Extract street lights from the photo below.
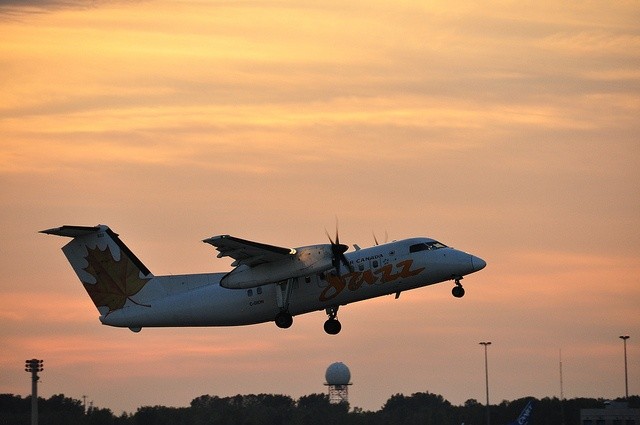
[618,334,631,400]
[478,340,495,425]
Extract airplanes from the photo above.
[37,221,488,337]
